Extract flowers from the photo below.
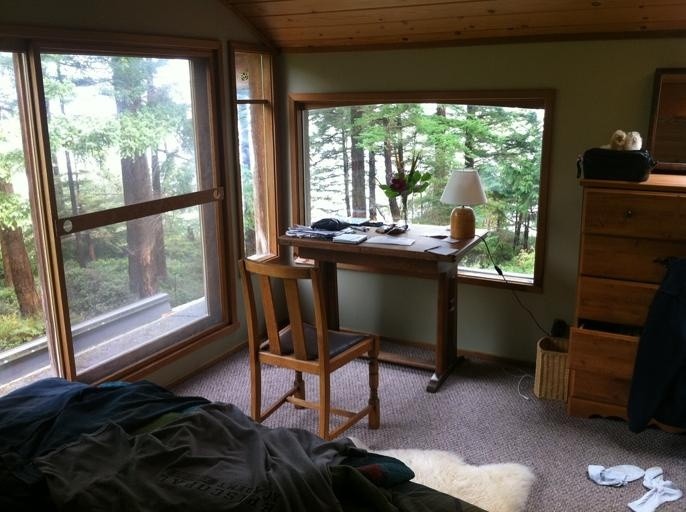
[378,167,432,227]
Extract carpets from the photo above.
[346,437,536,512]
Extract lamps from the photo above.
[439,169,487,241]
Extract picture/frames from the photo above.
[646,65,684,175]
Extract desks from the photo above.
[272,219,488,391]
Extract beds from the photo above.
[0,376,485,510]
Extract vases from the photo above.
[399,200,411,231]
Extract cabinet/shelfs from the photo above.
[563,171,685,435]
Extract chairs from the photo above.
[235,256,380,442]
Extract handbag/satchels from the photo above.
[311,218,349,231]
[577,147,656,182]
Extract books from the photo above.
[333,233,367,245]
[412,238,459,257]
